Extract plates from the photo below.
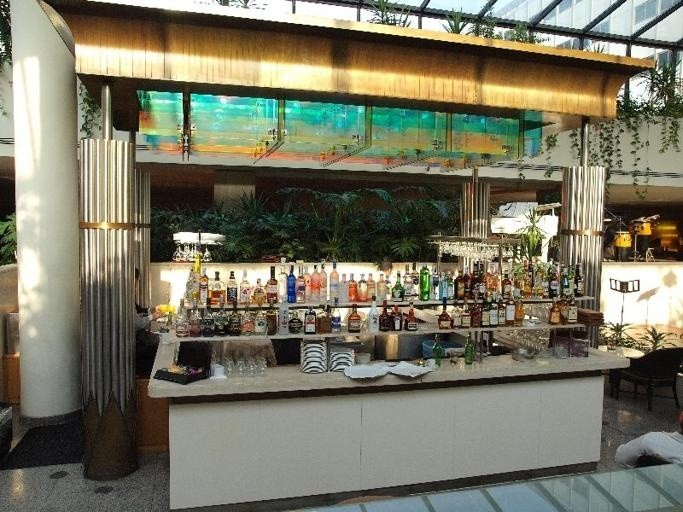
[344,361,432,379]
[300,341,356,374]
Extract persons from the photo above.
[614,413,683,469]
[135,266,164,378]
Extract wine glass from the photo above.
[438,242,513,257]
[172,241,213,262]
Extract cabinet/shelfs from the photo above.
[149,297,630,401]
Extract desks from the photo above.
[597,346,645,398]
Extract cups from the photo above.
[229,355,267,379]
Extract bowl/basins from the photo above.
[356,352,370,363]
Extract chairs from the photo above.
[617,347,683,411]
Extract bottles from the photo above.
[432,333,475,367]
[176,258,583,338]
